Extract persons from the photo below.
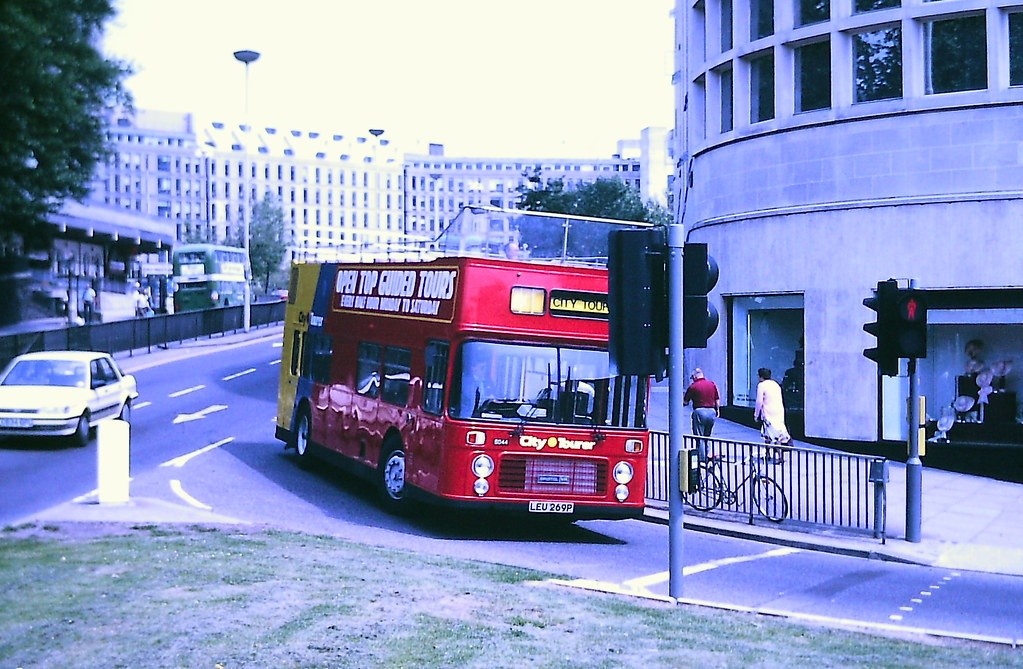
[460,357,499,417]
[81,284,96,326]
[682,368,720,465]
[503,244,521,261]
[754,367,793,464]
[137,286,152,318]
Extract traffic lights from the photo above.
[606,230,665,378]
[684,242,720,347]
[863,278,926,378]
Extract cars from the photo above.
[0,351,138,447]
[276,290,288,301]
[23,271,173,319]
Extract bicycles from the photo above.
[681,451,788,523]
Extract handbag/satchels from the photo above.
[774,436,794,452]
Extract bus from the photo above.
[275,257,650,525]
[174,244,252,307]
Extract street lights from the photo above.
[369,128,384,258]
[233,50,261,333]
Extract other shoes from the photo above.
[698,460,706,463]
[762,456,770,460]
[777,458,784,463]
